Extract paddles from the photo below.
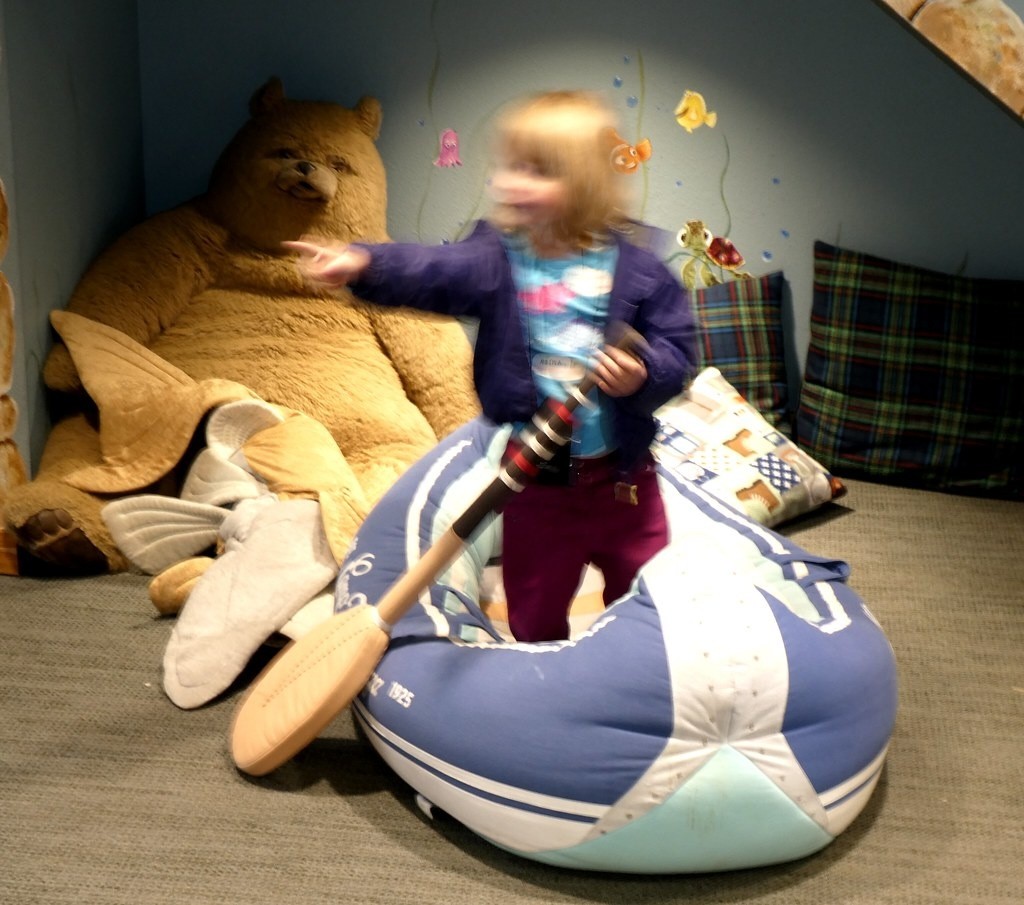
[227,329,655,778]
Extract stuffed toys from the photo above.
[9,81,486,710]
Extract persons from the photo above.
[280,91,700,644]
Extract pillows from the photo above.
[650,363,846,531]
[794,240,1024,502]
[676,272,792,444]
